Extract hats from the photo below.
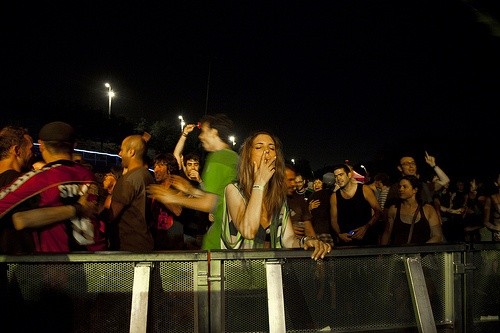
[323,172,337,185]
[37,121,76,144]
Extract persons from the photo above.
[0,112,241,333]
[219,133,331,333]
[284,151,500,333]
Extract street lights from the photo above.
[361,165,368,182]
[105,82,119,144]
[178,115,186,133]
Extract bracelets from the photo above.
[432,165,437,168]
[303,235,316,240]
[252,185,264,191]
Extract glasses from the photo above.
[401,160,416,166]
